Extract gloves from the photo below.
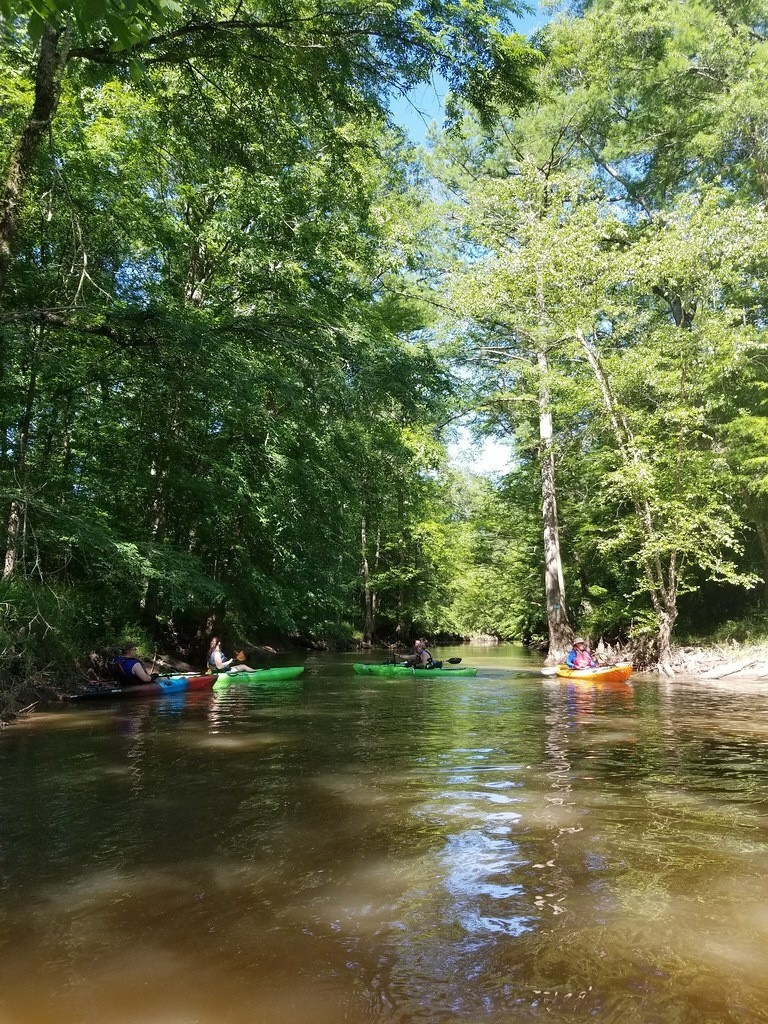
[149,673,159,682]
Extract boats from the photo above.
[159,665,307,683]
[352,662,479,678]
[68,674,220,702]
[556,661,636,682]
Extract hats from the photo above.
[573,638,585,644]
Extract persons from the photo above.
[207,636,256,673]
[113,642,159,685]
[565,637,600,670]
[393,638,432,669]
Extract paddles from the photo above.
[158,672,204,678]
[233,650,248,662]
[538,661,634,676]
[435,657,463,664]
